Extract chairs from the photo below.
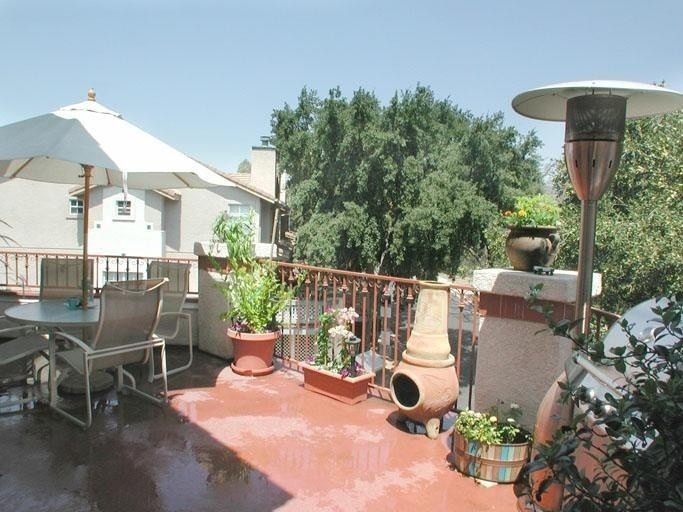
[0,259,195,429]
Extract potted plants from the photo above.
[452,395,535,485]
[212,206,312,379]
[298,307,377,407]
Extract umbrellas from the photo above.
[0,87,240,342]
